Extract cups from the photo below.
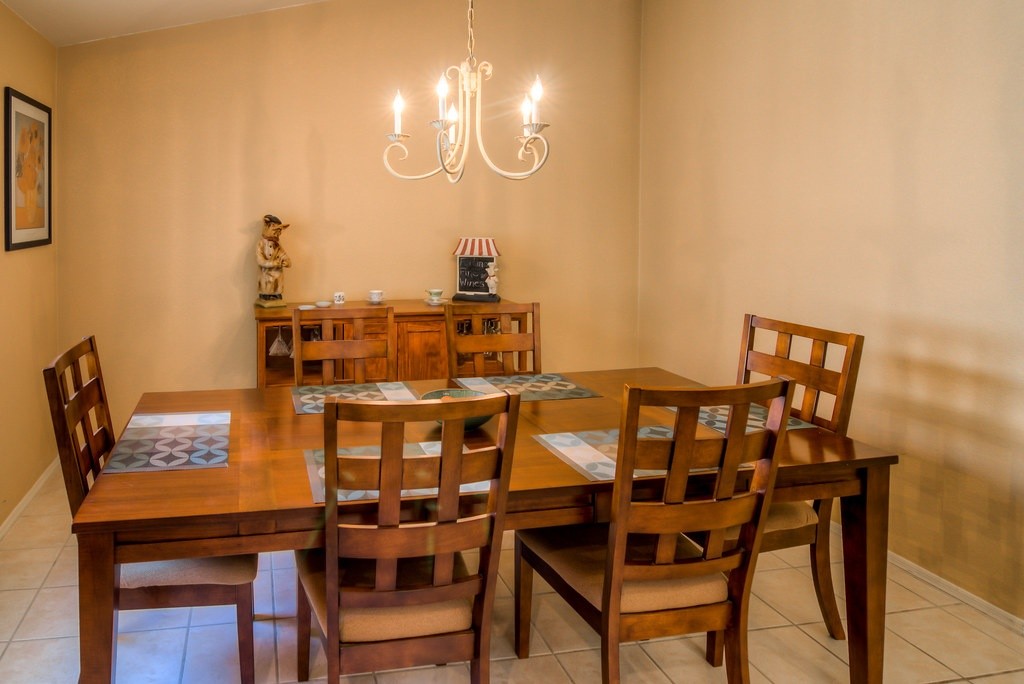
[429,289,443,302]
[370,290,383,300]
[334,291,345,303]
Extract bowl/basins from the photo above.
[420,389,495,429]
[316,301,332,307]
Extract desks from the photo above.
[72,362,899,683]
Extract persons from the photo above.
[256,215,291,301]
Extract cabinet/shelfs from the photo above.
[255,295,528,388]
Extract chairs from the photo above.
[291,306,396,386]
[706,312,864,669]
[515,373,796,682]
[43,333,257,683]
[295,386,521,682]
[444,303,542,377]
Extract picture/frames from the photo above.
[3,85,52,252]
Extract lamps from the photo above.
[384,0,558,182]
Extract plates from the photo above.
[425,299,448,306]
[369,300,383,304]
